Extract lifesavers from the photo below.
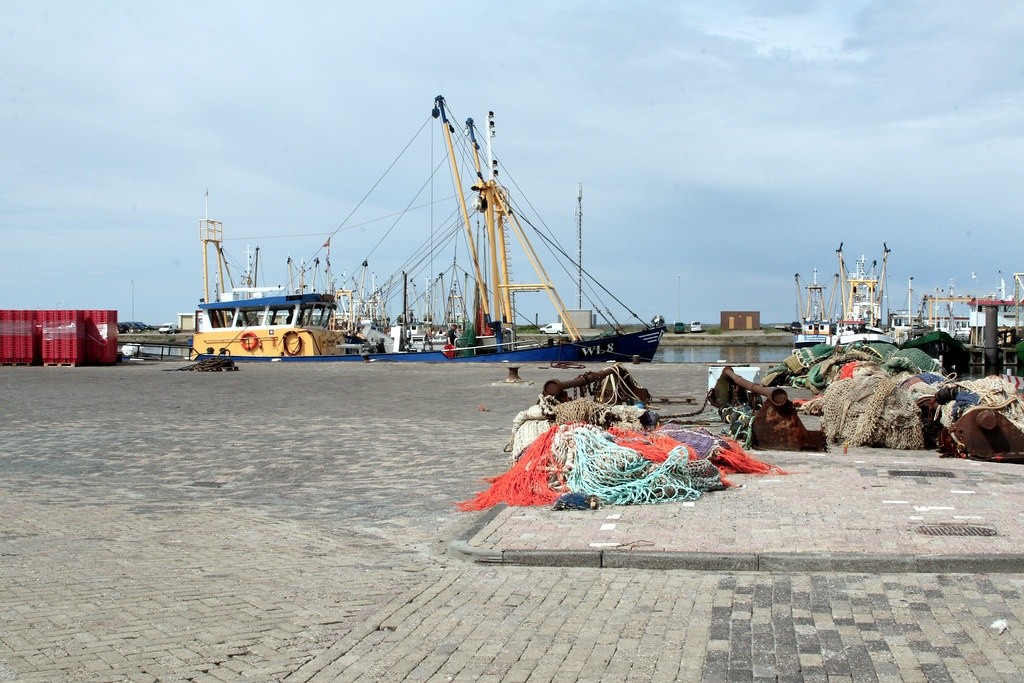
[240,332,259,351]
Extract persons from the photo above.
[447,324,457,345]
[286,309,300,324]
[1003,328,1019,362]
[252,317,259,326]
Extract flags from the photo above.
[323,237,330,247]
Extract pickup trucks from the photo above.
[690,321,702,332]
[673,322,686,334]
[158,323,179,334]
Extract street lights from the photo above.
[129,279,136,323]
[677,274,681,322]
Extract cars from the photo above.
[118,321,150,334]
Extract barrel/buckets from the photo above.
[445,345,455,358]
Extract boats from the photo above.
[188,94,671,364]
[792,241,1024,349]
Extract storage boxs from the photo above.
[708,367,760,405]
[0,309,118,364]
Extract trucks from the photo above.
[538,323,564,333]
